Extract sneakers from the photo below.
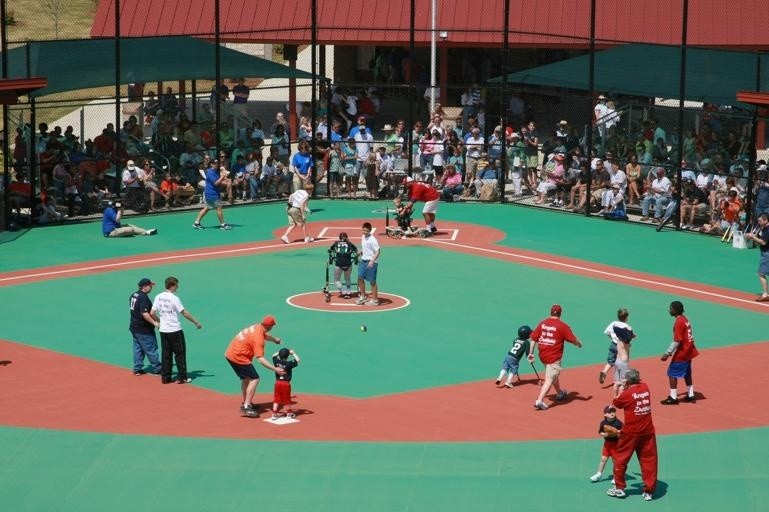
[304,236,315,243]
[534,389,569,410]
[146,228,156,235]
[193,221,203,230]
[598,370,607,384]
[356,296,380,307]
[431,227,437,233]
[589,469,656,502]
[755,294,769,302]
[495,377,515,388]
[270,411,298,420]
[280,234,291,244]
[132,365,193,384]
[239,402,262,418]
[343,294,351,299]
[220,224,231,231]
[421,230,432,236]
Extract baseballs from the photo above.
[361,325,366,332]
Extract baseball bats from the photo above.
[721,226,730,242]
[657,215,671,230]
[727,226,736,242]
[744,224,761,234]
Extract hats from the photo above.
[506,114,768,233]
[61,113,504,213]
[137,277,156,288]
[262,315,276,327]
[604,404,617,415]
[597,96,608,101]
[550,304,563,314]
[517,325,533,338]
[279,348,290,357]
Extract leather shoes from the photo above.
[683,394,698,403]
[660,395,680,405]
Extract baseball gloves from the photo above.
[604,425,620,438]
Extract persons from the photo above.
[598,308,635,384]
[0,47,769,236]
[224,315,287,418]
[129,278,163,376]
[744,213,769,301]
[272,348,300,421]
[590,405,624,485]
[606,368,658,501]
[149,276,202,384]
[613,327,632,399]
[282,186,315,244]
[328,223,379,305]
[495,326,533,388]
[660,300,700,405]
[526,304,582,410]
